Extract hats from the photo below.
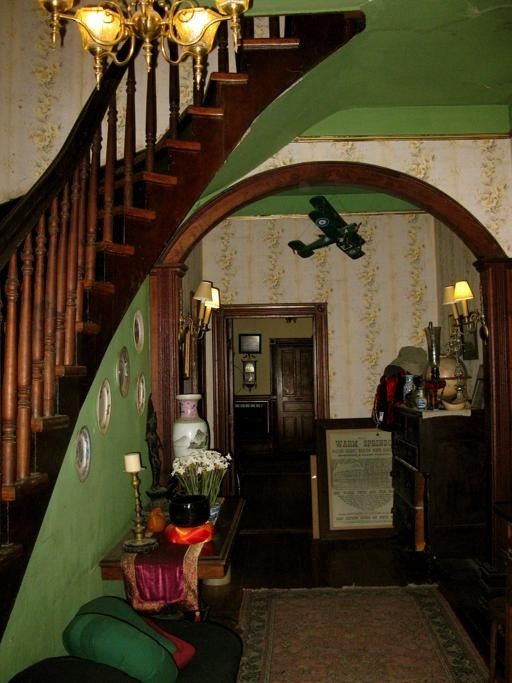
[390,347,426,377]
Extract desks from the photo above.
[235,394,278,449]
[100,497,247,639]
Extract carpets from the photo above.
[236,582,496,682]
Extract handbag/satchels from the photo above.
[383,365,409,402]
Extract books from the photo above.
[400,404,472,420]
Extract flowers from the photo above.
[170,448,232,507]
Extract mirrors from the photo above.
[241,354,257,393]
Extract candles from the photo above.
[124,452,142,472]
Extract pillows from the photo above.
[76,595,177,655]
[144,616,197,669]
[61,612,179,683]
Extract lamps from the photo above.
[442,282,489,345]
[36,0,251,92]
[178,281,221,344]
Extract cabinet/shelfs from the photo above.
[394,405,490,583]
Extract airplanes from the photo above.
[288,196,366,260]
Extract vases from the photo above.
[426,355,467,401]
[402,374,416,407]
[416,386,427,410]
[207,506,221,526]
[172,393,210,456]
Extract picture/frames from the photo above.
[314,418,395,542]
[447,312,478,361]
[238,333,262,355]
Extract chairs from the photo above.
[8,621,244,682]
